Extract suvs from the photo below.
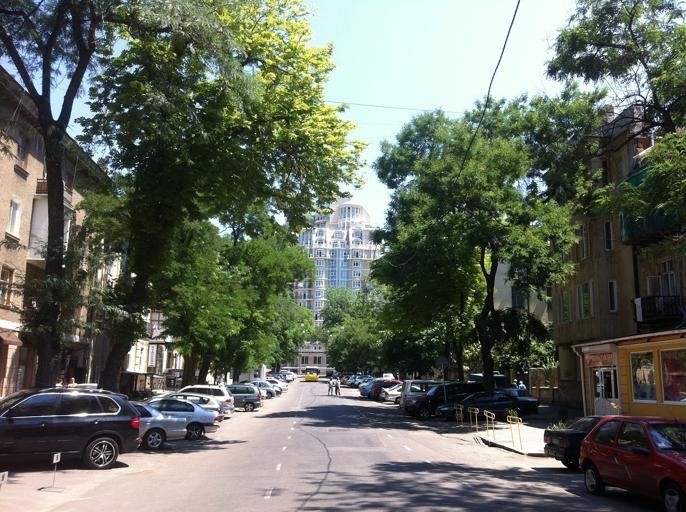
[0,379,144,470]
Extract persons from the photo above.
[328,377,334,396]
[519,380,526,396]
[510,378,518,396]
[335,377,340,395]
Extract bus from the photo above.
[304,365,320,383]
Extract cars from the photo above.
[536,413,610,474]
[336,372,537,424]
[572,414,686,512]
[129,370,296,450]
